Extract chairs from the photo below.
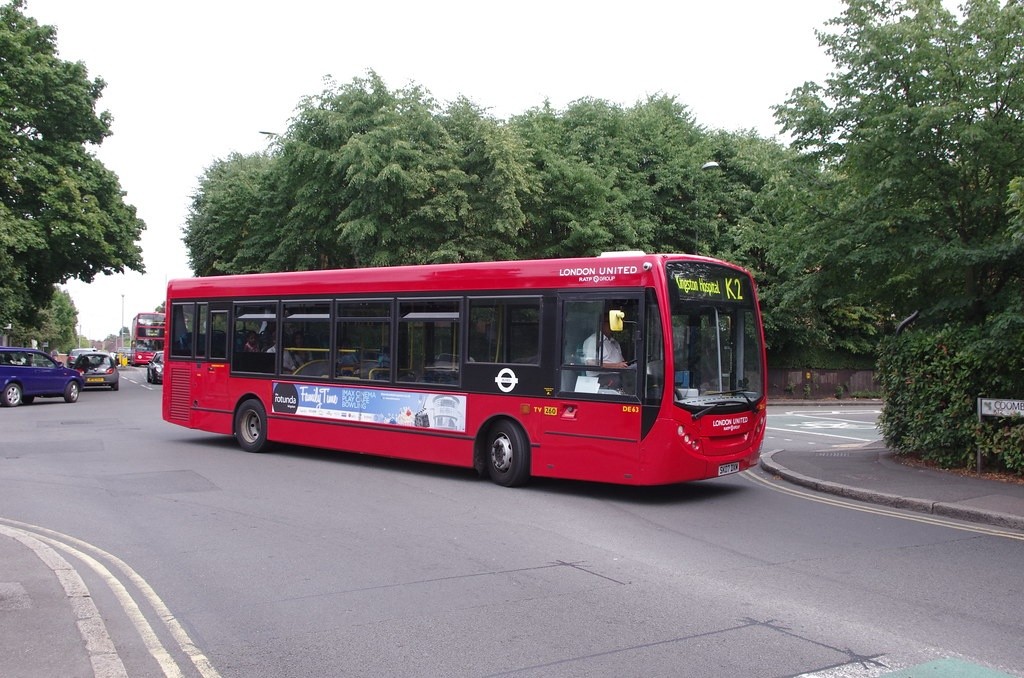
[4,355,14,363]
[180,328,356,377]
[82,359,89,367]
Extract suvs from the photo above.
[0,346,86,407]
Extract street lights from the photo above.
[694,160,719,254]
[121,294,125,348]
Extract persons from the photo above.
[583,313,628,387]
[51,348,59,361]
[27,354,33,365]
[244,331,390,376]
[138,340,154,351]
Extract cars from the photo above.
[115,346,132,363]
[66,347,120,393]
[146,350,164,384]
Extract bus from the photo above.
[161,252,769,487]
[132,312,166,367]
